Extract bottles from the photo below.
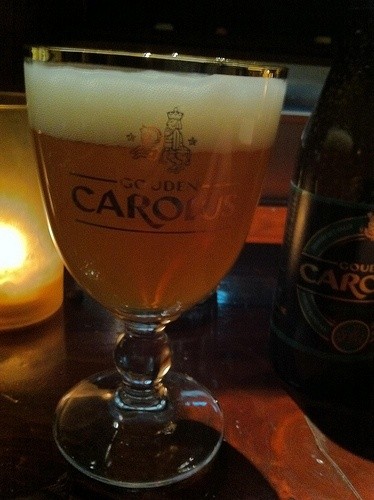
[270,1,374,404]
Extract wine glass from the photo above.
[24,44,288,487]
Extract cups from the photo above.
[1,93,63,331]
